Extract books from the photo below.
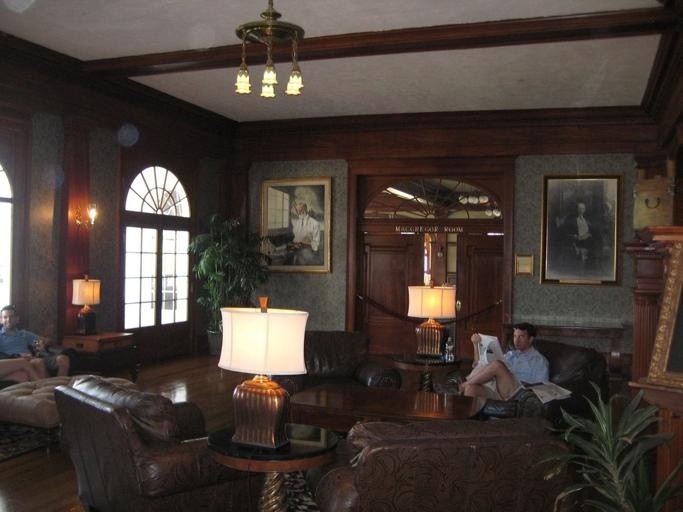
[285,422,327,448]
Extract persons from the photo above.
[458,323,549,413]
[565,202,596,263]
[292,202,321,265]
[0,358,39,382]
[0,306,70,379]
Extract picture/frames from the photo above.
[539,173,624,287]
[259,177,332,273]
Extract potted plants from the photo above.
[189,215,272,356]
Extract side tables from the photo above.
[205,423,340,512]
[384,356,462,390]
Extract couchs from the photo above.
[320,419,578,512]
[0,323,79,392]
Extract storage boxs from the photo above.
[631,177,674,230]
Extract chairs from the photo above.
[275,328,403,388]
[54,377,267,512]
[445,338,608,437]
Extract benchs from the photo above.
[0,374,138,442]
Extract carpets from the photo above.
[0,397,60,462]
[251,467,320,512]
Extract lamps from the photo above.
[406,285,457,355]
[218,295,309,449]
[74,202,98,225]
[235,0,304,98]
[72,273,101,337]
[459,191,501,217]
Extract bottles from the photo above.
[444,337,454,361]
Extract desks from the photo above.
[62,331,138,383]
[628,377,683,512]
